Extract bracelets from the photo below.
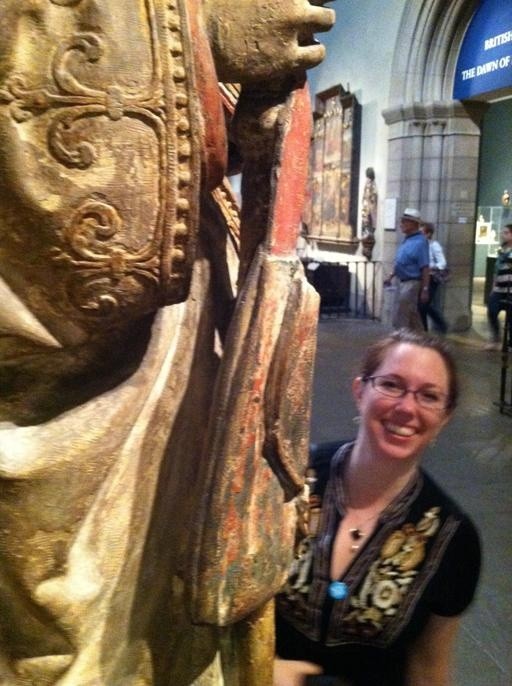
[389,272,394,277]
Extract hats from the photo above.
[399,208,425,225]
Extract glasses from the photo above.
[361,374,456,414]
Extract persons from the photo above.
[477,224,512,353]
[265,324,483,684]
[296,220,309,259]
[381,207,432,333]
[418,220,450,339]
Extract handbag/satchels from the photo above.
[431,265,455,286]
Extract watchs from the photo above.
[421,285,428,291]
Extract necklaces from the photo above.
[329,437,425,560]
